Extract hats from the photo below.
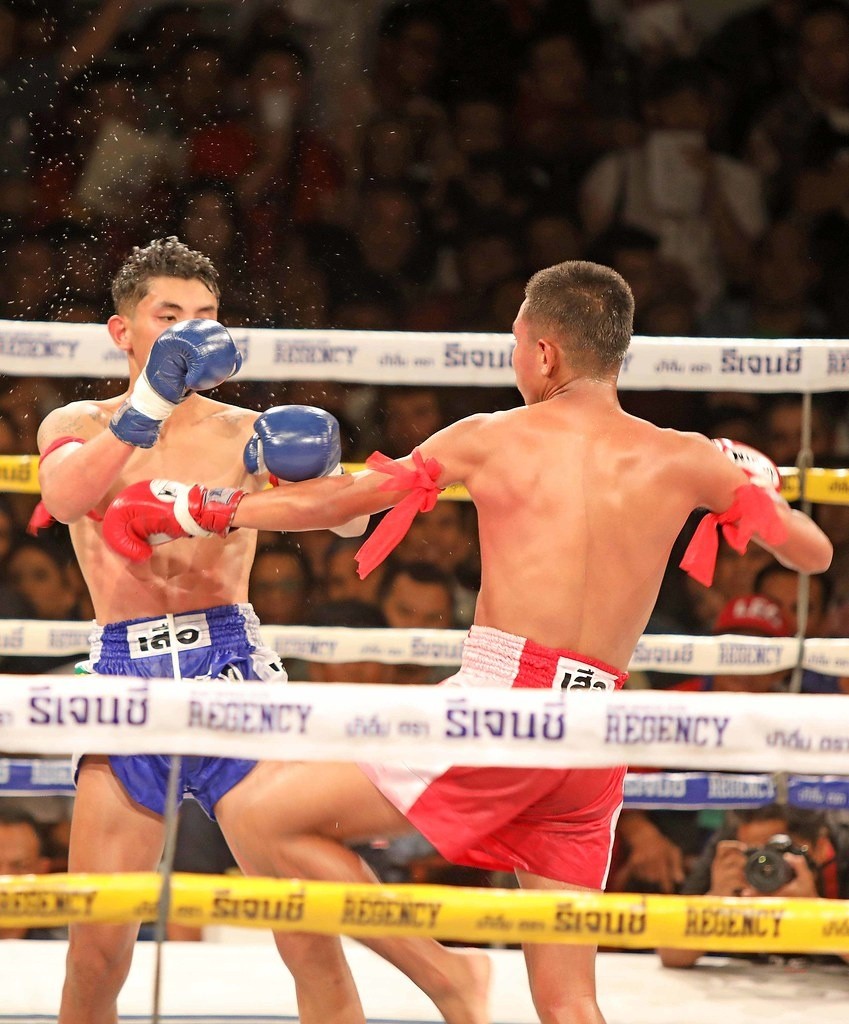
[713,593,788,635]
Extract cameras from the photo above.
[740,833,816,892]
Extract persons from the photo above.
[0,0,849,969]
[103,261,834,1024]
[36,236,371,1024]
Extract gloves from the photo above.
[110,319,242,449]
[713,437,784,496]
[102,478,249,560]
[242,404,344,484]
